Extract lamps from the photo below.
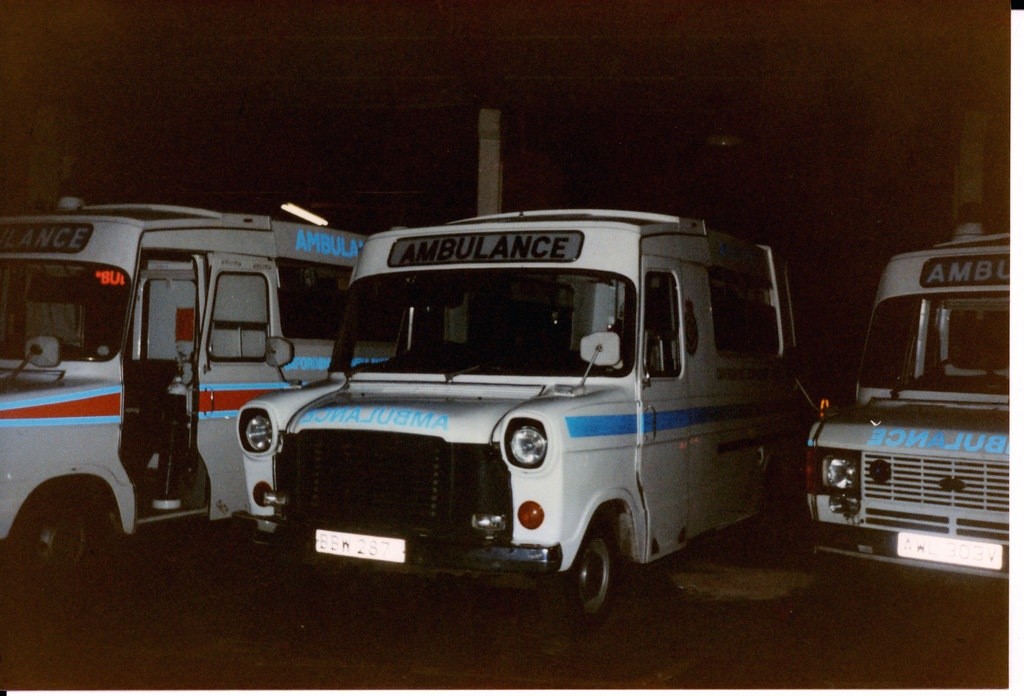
[280,202,329,227]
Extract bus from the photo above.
[233,204,820,622]
[798,223,1006,587]
[2,195,401,611]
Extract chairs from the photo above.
[953,310,1009,371]
